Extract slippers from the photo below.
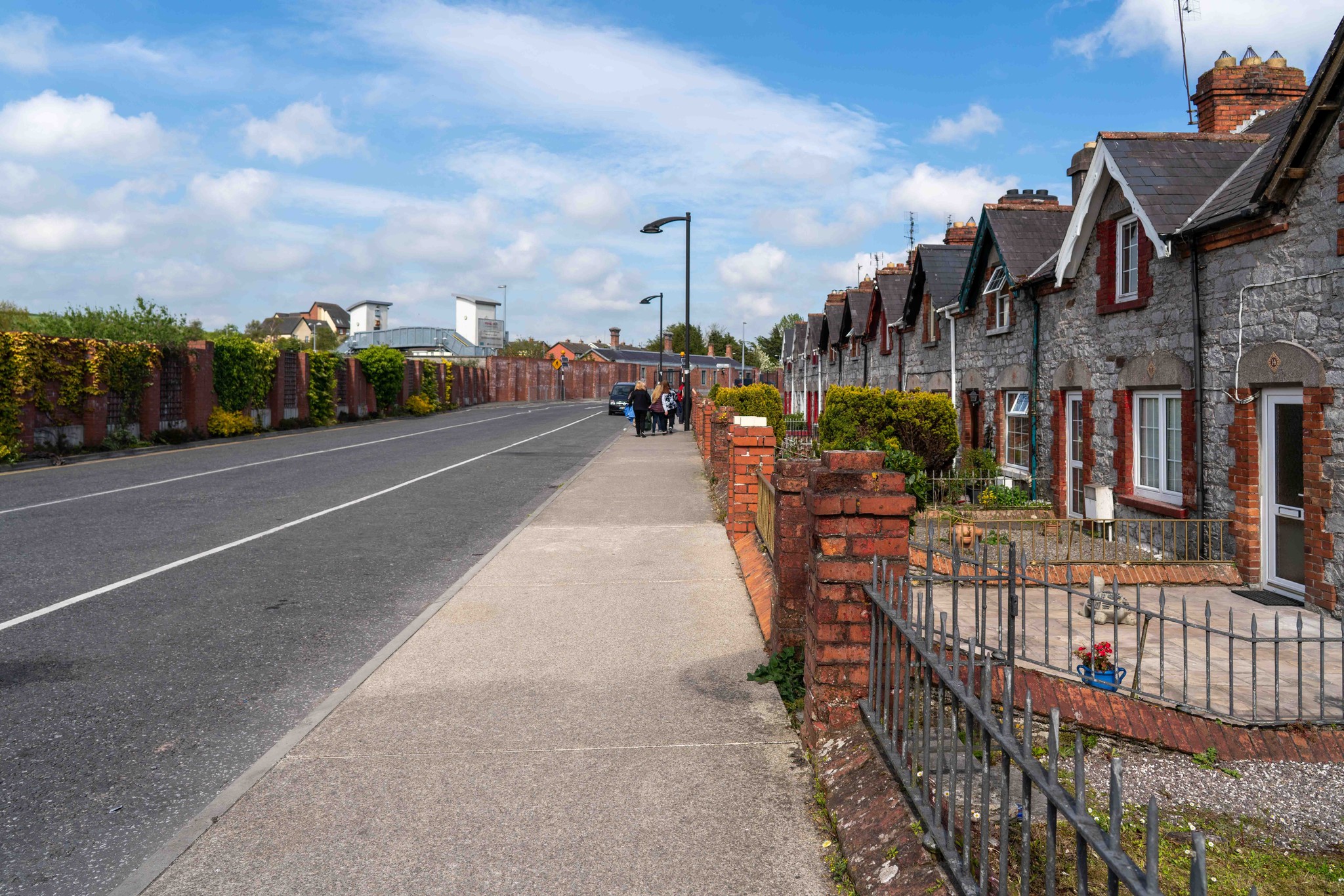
[662,430,668,435]
[652,434,655,436]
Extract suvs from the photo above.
[608,382,636,416]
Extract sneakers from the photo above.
[669,427,673,433]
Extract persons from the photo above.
[628,380,678,438]
[677,381,697,424]
[640,376,646,383]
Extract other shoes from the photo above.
[657,429,662,432]
[640,432,646,438]
[635,434,640,437]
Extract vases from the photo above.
[1077,663,1126,693]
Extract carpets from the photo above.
[1231,590,1304,606]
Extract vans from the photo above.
[735,378,752,388]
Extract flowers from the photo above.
[1074,641,1112,672]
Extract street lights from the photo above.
[639,292,663,382]
[741,321,747,387]
[774,354,781,368]
[639,211,691,430]
